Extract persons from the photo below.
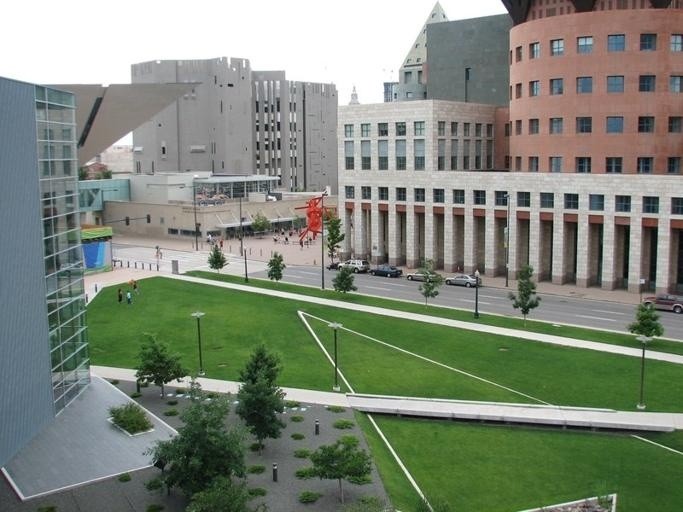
[207,232,224,250]
[273,226,312,248]
[117,279,139,304]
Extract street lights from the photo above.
[239,193,248,282]
[504,192,511,288]
[321,191,328,288]
[193,182,198,252]
[636,335,653,409]
[475,268,480,319]
[191,310,206,376]
[328,322,343,392]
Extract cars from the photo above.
[338,259,482,288]
[643,293,683,314]
